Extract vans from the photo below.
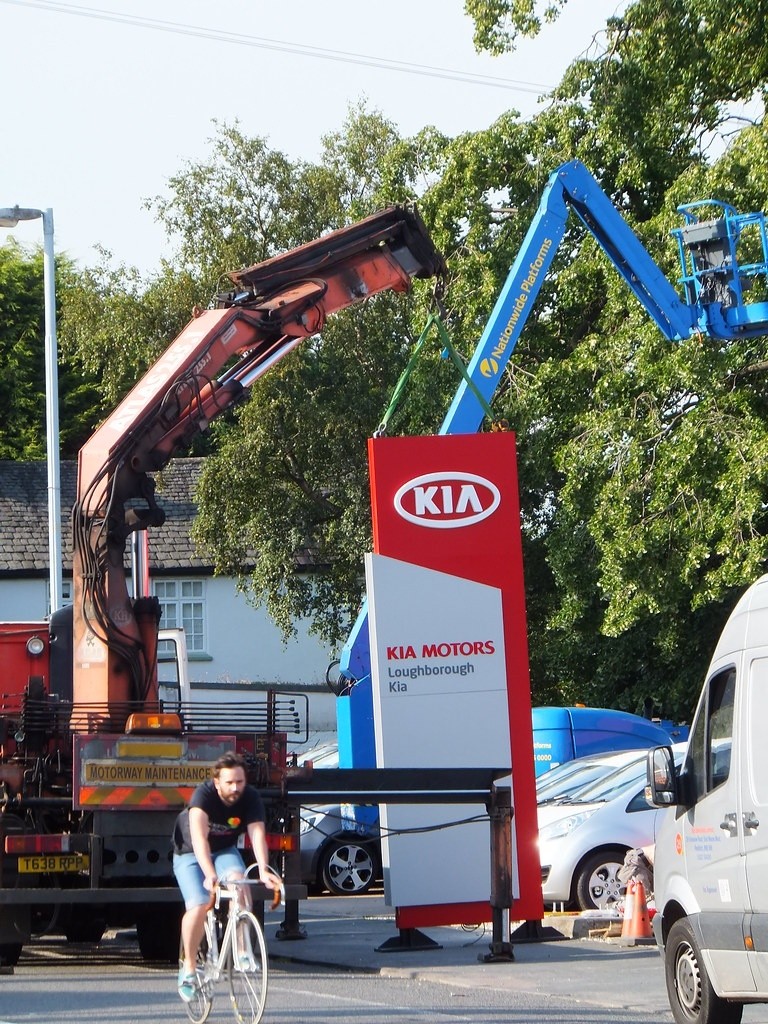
[644,573,767,1024]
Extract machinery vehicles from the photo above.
[325,151,767,845]
[0,200,458,977]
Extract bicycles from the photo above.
[179,862,285,1024]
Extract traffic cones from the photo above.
[605,878,659,947]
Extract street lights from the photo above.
[0,204,66,615]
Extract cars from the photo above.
[291,740,384,898]
[533,733,736,911]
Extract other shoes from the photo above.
[234,953,260,973]
[177,967,198,1003]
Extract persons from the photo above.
[170,749,283,1003]
